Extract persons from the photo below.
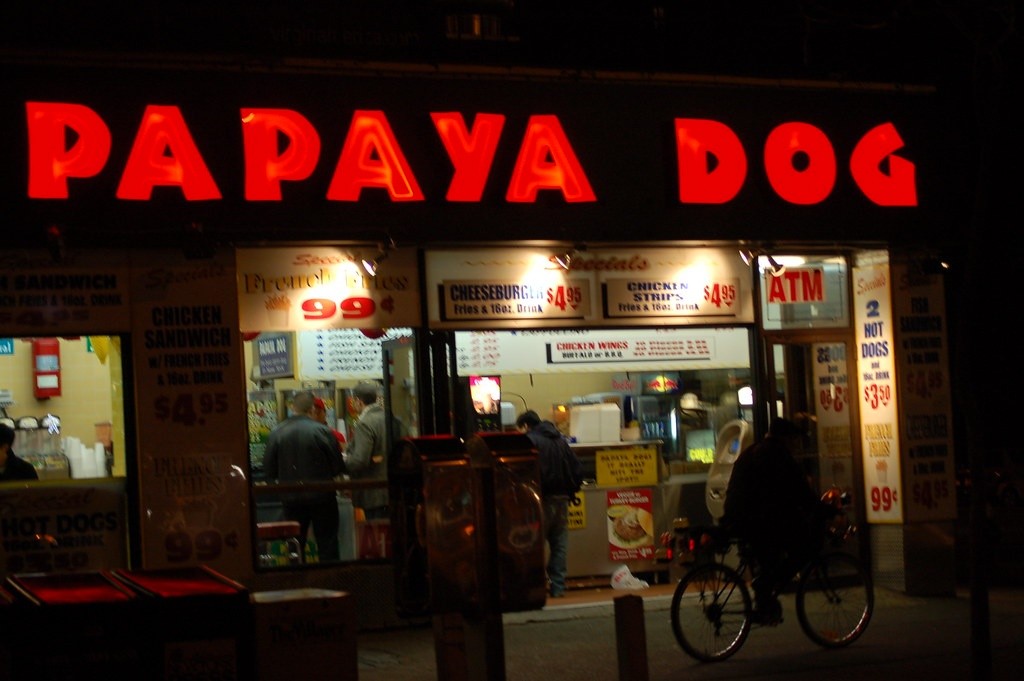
[515,411,576,599]
[261,392,346,566]
[0,423,38,484]
[312,396,345,453]
[89,419,116,477]
[722,417,843,627]
[341,382,407,520]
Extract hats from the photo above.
[769,417,803,437]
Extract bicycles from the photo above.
[668,489,876,664]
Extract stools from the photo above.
[255,522,302,566]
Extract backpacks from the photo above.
[527,429,584,495]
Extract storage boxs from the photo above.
[251,588,356,681]
